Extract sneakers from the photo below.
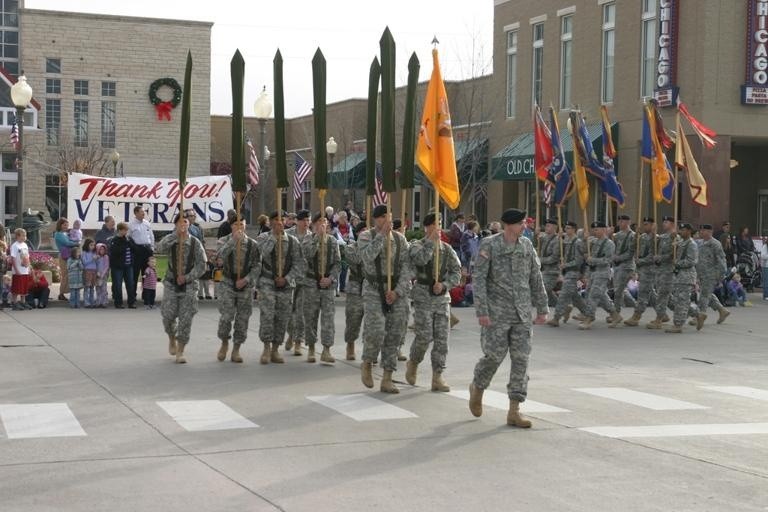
[1,295,157,312]
[398,350,407,362]
[740,301,753,307]
[197,294,213,301]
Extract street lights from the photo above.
[10,68,35,228]
[326,136,338,207]
[110,150,121,176]
[263,145,271,216]
[567,116,586,227]
[252,85,272,218]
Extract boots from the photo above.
[430,371,452,392]
[260,341,283,363]
[405,359,419,386]
[217,339,229,361]
[360,358,377,388]
[506,398,533,428]
[469,384,482,417]
[380,368,401,395]
[285,335,317,363]
[320,345,335,363]
[347,342,356,360]
[232,344,244,363]
[168,335,188,363]
[547,305,731,332]
[406,312,459,330]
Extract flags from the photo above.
[10,116,19,149]
[415,46,461,210]
[533,93,711,209]
[292,152,312,201]
[373,161,388,208]
[249,145,260,188]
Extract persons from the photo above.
[155,207,207,362]
[355,205,463,392]
[446,220,499,309]
[521,215,758,333]
[206,207,367,362]
[0,203,155,310]
[468,208,549,426]
[761,243,768,301]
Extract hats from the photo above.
[543,215,713,230]
[371,203,392,219]
[312,211,327,224]
[269,208,286,221]
[501,208,528,225]
[392,220,401,230]
[422,213,441,227]
[526,217,536,223]
[229,213,247,225]
[296,209,311,219]
[355,221,366,234]
[174,212,189,224]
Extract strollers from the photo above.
[735,250,762,293]
